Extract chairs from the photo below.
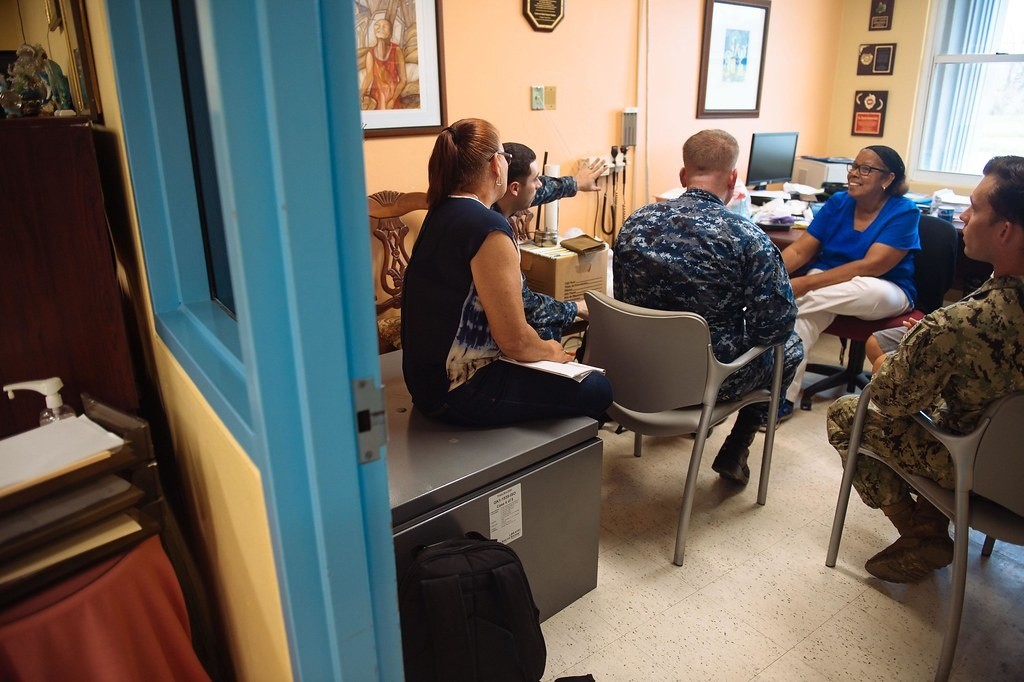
[799,215,960,410]
[575,289,787,567]
[824,381,1024,682]
[367,191,462,350]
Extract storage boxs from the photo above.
[516,237,609,304]
[379,348,604,624]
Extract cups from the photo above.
[938,207,954,223]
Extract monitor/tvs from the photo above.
[744,132,798,191]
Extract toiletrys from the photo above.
[2,376,78,427]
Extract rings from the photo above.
[587,166,593,170]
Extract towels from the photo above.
[0,533,212,682]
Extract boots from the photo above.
[712,411,761,485]
[880,498,919,537]
[865,494,954,583]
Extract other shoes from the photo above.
[758,401,796,432]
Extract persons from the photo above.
[761,145,923,426]
[613,129,804,486]
[398,117,614,430]
[825,155,1024,584]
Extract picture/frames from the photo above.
[355,0,448,138]
[697,0,772,119]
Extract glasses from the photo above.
[847,162,890,176]
[487,151,512,166]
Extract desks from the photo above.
[653,190,995,293]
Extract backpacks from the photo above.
[395,530,547,682]
